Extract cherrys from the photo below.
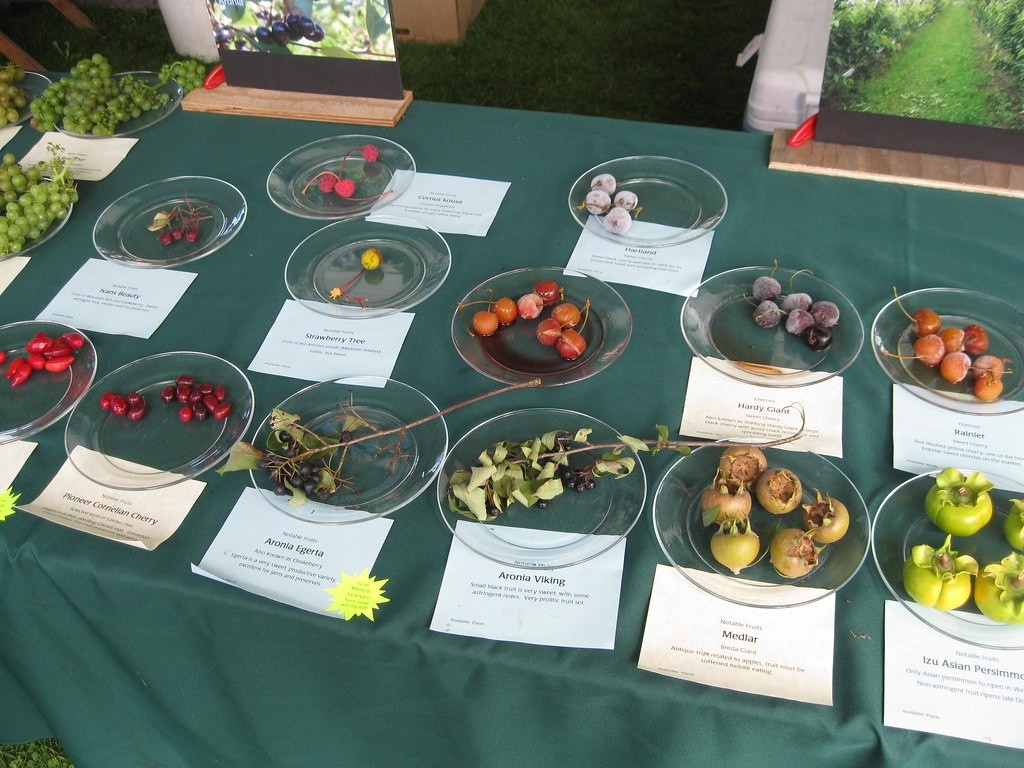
[577,175,643,235]
[881,285,1012,401]
[159,205,199,246]
[205,0,324,46]
[457,281,590,360]
[743,259,840,351]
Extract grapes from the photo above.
[30,53,207,136]
[0,146,81,257]
[0,61,27,128]
[101,376,232,422]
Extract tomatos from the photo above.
[0,331,86,388]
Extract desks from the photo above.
[0,70,1024,768]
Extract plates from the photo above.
[0,71,52,128]
[285,215,452,319]
[267,135,416,220]
[681,265,864,389]
[93,176,247,269]
[437,407,647,570]
[653,438,870,608]
[0,179,73,260]
[568,155,728,248]
[870,288,1024,416]
[64,351,255,491]
[452,265,632,389]
[54,71,183,139]
[872,468,1024,649]
[250,374,449,525]
[0,321,98,445]
